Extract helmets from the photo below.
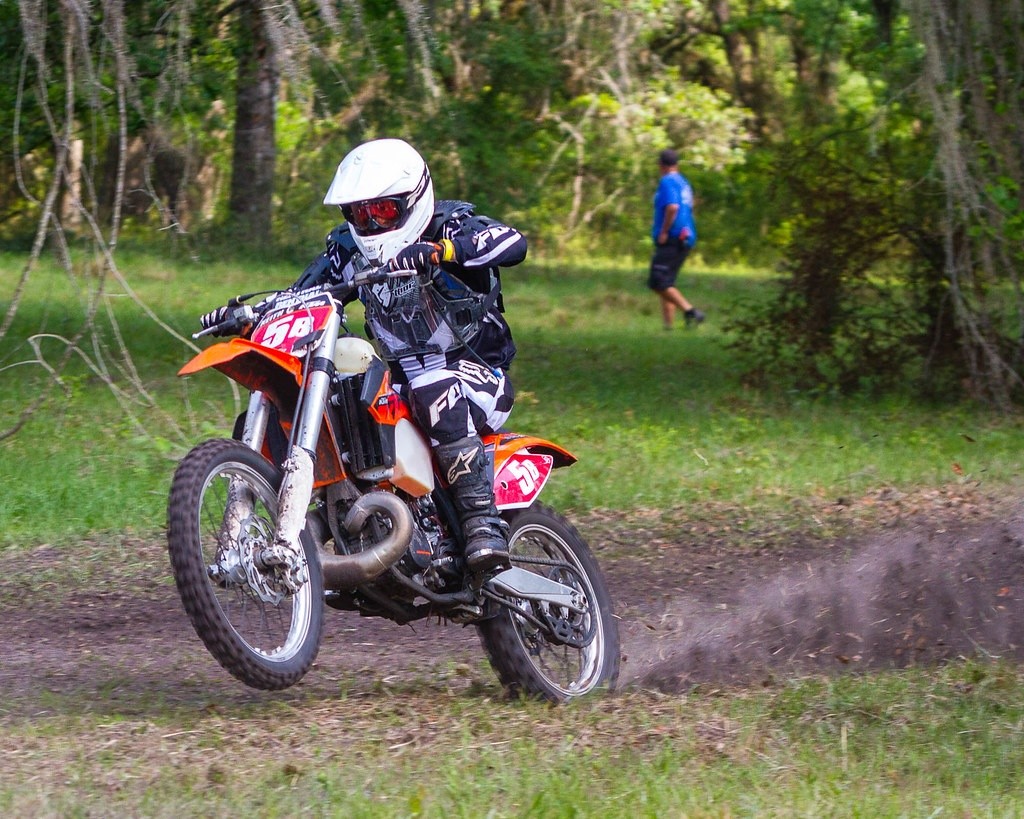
[324,138,434,269]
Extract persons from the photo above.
[647,149,705,331]
[199,138,528,609]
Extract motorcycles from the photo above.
[163,250,622,713]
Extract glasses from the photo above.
[341,197,409,236]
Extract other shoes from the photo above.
[685,308,704,329]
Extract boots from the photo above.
[325,539,358,612]
[433,434,511,573]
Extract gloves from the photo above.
[199,303,244,338]
[394,242,442,282]
